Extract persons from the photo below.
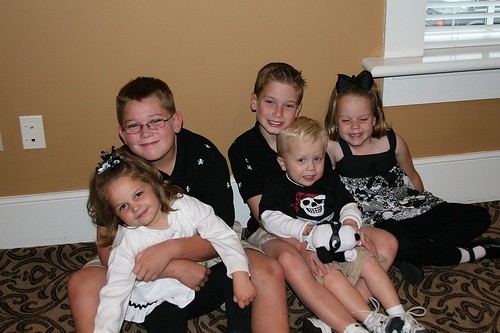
[258,115,430,333]
[66,75,289,333]
[87,147,258,332]
[324,68,499,284]
[227,62,398,331]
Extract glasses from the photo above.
[121,115,173,134]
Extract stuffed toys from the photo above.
[313,219,361,264]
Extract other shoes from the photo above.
[459,243,492,263]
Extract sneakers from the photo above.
[389,306,429,333]
[351,297,412,333]
[306,318,332,333]
